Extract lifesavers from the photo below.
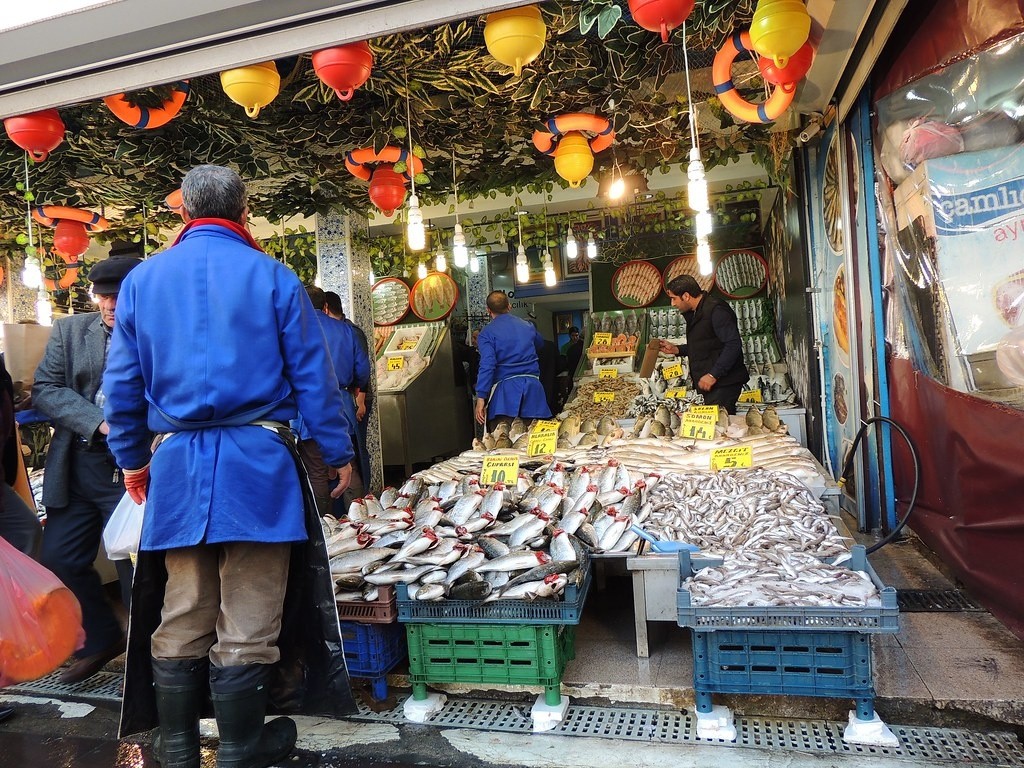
[31,206,109,231]
[165,188,183,213]
[532,112,616,157]
[36,244,77,288]
[344,145,423,184]
[105,80,191,128]
[712,33,796,121]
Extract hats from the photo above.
[89,256,144,294]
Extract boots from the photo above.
[150,656,202,768]
[210,661,297,768]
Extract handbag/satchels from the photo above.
[0,537,84,688]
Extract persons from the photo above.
[561,326,586,372]
[659,275,750,415]
[464,289,552,433]
[290,285,373,519]
[30,256,146,684]
[0,354,42,557]
[102,164,354,768]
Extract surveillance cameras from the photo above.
[528,312,537,319]
[800,122,821,142]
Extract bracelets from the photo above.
[709,374,713,378]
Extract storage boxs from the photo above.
[406,624,573,705]
[340,622,405,699]
[686,627,879,717]
[384,326,434,358]
[592,355,635,374]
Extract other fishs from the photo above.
[665,255,712,291]
[373,282,408,323]
[617,264,662,305]
[321,296,883,628]
[29,466,47,516]
[414,275,453,318]
[716,253,766,293]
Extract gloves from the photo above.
[124,466,149,505]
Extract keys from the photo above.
[111,465,120,483]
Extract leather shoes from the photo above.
[59,631,127,683]
[0,707,15,721]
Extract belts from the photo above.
[72,437,108,451]
